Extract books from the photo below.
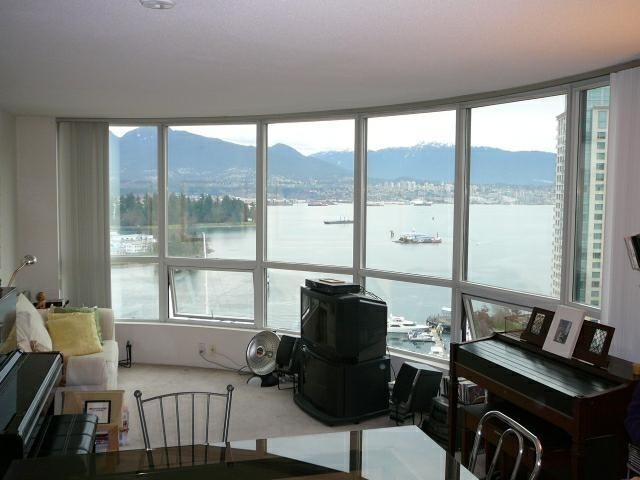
[442,374,487,404]
[92,406,129,450]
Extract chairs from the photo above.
[134,385,235,449]
[0,308,119,415]
[468,410,543,480]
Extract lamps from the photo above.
[8,255,40,287]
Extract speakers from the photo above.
[276,334,300,374]
[392,362,443,412]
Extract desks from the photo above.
[447,330,633,480]
[7,425,480,480]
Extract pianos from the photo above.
[1,348,63,479]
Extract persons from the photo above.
[34,292,46,310]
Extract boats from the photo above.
[407,321,443,343]
[414,201,433,206]
[392,231,443,245]
[384,312,432,340]
[426,303,454,335]
[324,216,356,226]
[368,200,385,206]
[263,192,351,208]
[425,322,450,359]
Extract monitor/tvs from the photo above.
[300,287,387,365]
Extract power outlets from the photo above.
[199,343,207,353]
[208,344,216,352]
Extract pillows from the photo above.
[16,291,104,358]
